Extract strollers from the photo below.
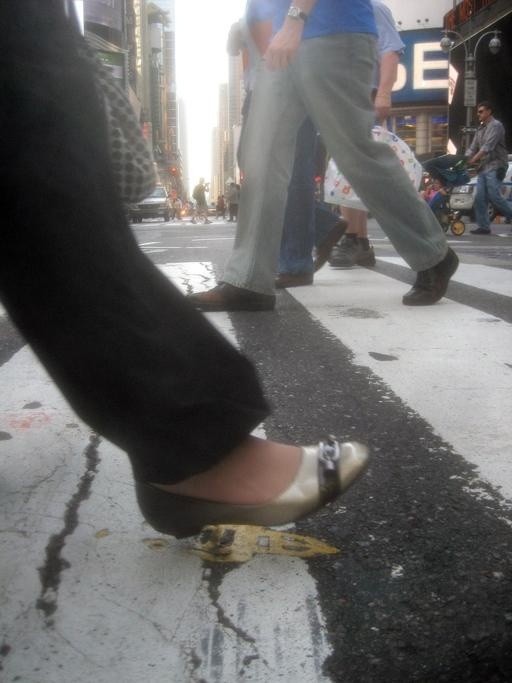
[421,153,480,236]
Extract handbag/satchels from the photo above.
[75,35,156,206]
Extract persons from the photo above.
[1,0,375,545]
[324,0,407,267]
[184,1,461,311]
[465,98,512,236]
[160,165,243,227]
[421,176,448,203]
[223,11,351,290]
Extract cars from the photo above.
[449,154,512,223]
[131,182,172,223]
[207,205,217,215]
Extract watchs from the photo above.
[284,3,308,23]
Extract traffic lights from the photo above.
[171,167,177,172]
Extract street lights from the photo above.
[440,30,502,155]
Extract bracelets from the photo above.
[377,90,390,98]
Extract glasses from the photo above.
[477,109,488,112]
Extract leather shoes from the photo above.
[275,271,314,286]
[185,283,277,312]
[313,218,346,272]
[401,250,459,304]
[134,437,370,541]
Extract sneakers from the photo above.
[330,235,375,267]
[470,227,491,235]
